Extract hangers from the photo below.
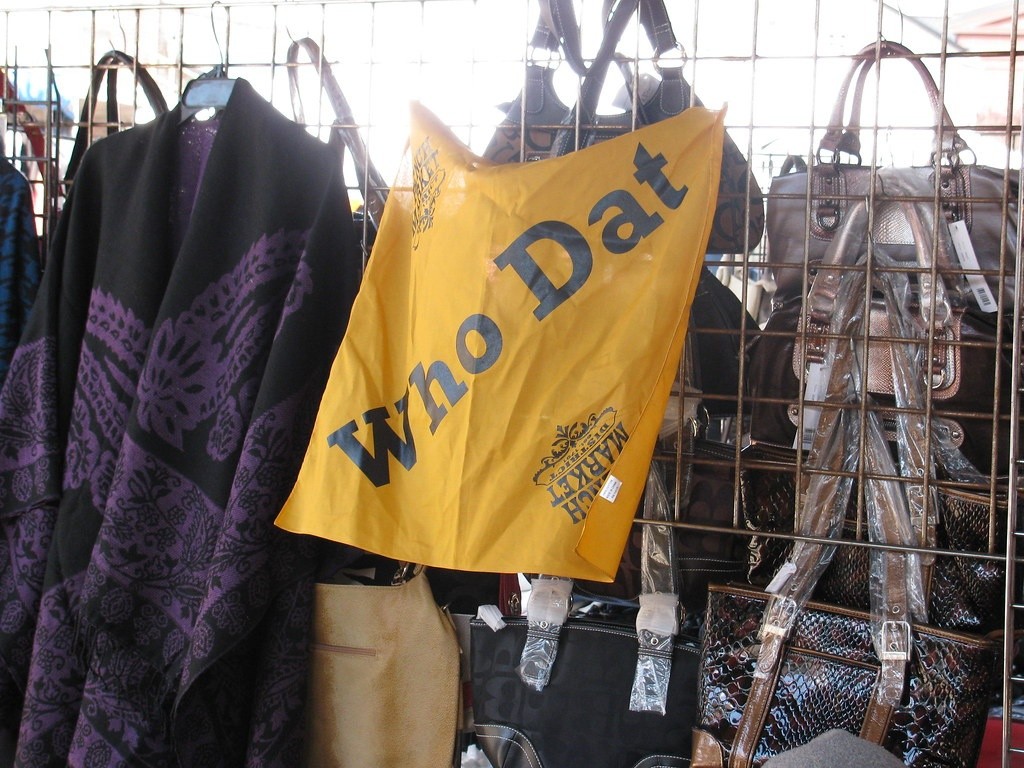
[169,1,239,127]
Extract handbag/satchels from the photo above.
[461,0,1024,768]
